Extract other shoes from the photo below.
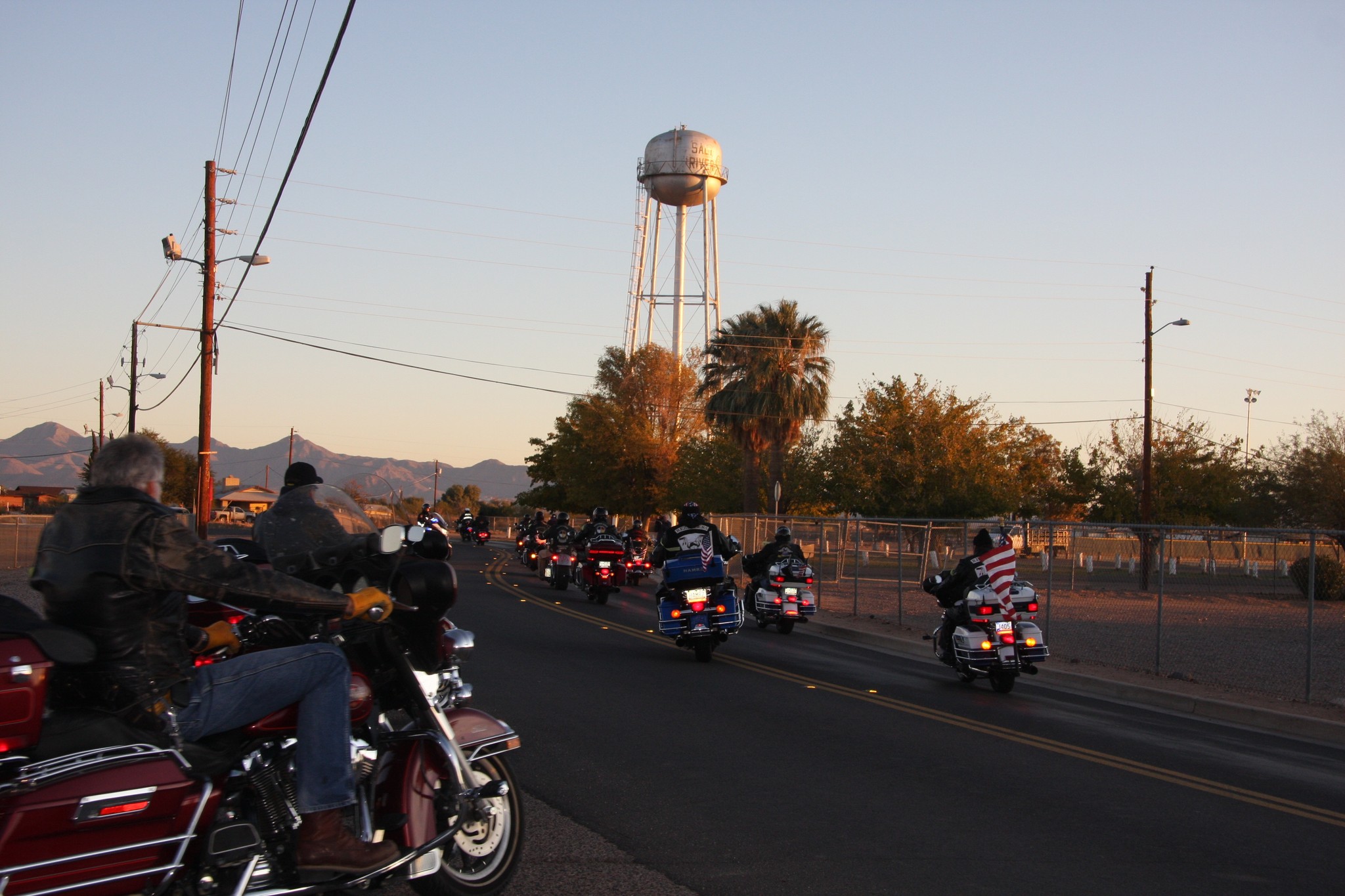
[574,580,582,589]
[938,653,955,666]
[535,570,545,581]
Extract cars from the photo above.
[169,507,190,515]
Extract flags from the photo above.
[701,530,714,572]
[978,533,1017,621]
[792,566,808,577]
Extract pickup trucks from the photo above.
[211,505,257,523]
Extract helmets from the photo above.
[633,520,642,528]
[551,514,556,518]
[465,508,470,513]
[593,507,608,519]
[682,502,701,518]
[556,512,570,525]
[535,511,544,521]
[775,526,791,541]
[524,514,531,521]
[422,504,430,513]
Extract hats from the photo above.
[972,528,993,548]
[284,462,324,487]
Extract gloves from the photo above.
[929,585,938,594]
[192,620,240,656]
[341,587,393,624]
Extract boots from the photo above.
[295,806,400,873]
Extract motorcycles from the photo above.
[454,518,474,541]
[922,549,1051,695]
[737,549,818,635]
[647,533,745,664]
[473,519,492,546]
[1,469,653,896]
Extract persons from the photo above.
[252,461,348,574]
[458,508,491,539]
[743,526,805,614]
[930,528,994,666]
[418,503,433,524]
[30,435,400,884]
[649,502,738,605]
[515,506,671,588]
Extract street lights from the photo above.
[1139,265,1193,594]
[193,254,270,540]
[99,413,124,451]
[127,372,167,434]
